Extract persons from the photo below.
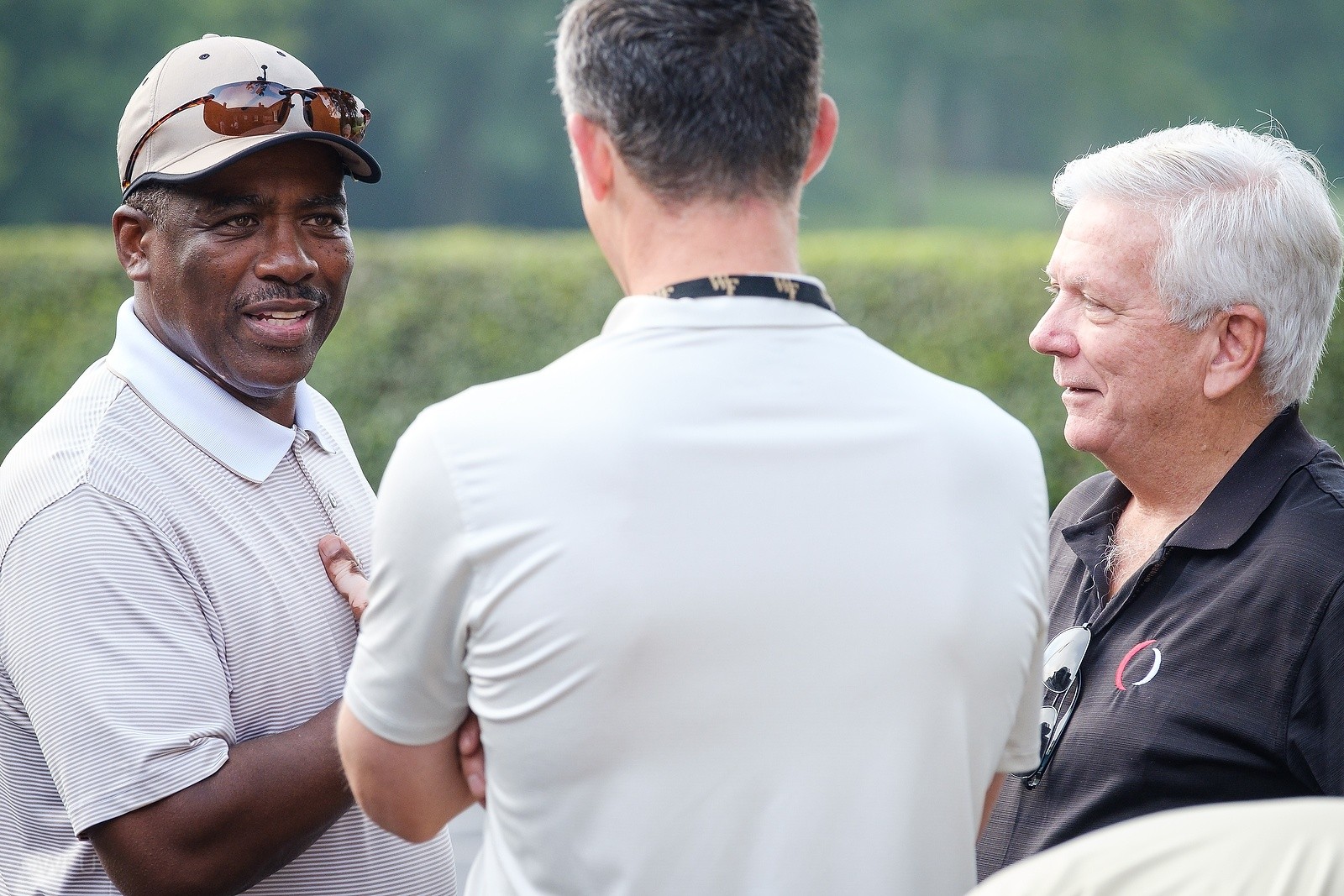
[338,0,1054,896]
[965,123,1344,896]
[1,34,458,896]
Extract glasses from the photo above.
[122,80,371,187]
[1010,622,1092,791]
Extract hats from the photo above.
[117,33,382,205]
